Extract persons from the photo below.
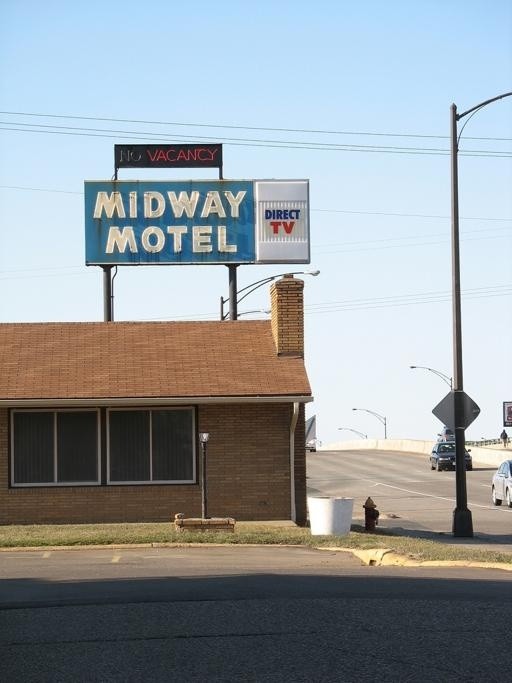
[500,428,508,447]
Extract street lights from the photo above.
[352,408,387,439]
[337,428,367,439]
[220,269,320,321]
[410,365,452,390]
[199,431,211,519]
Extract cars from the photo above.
[305,439,316,453]
[491,459,512,508]
[429,442,473,472]
[437,426,455,442]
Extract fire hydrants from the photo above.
[362,497,379,531]
